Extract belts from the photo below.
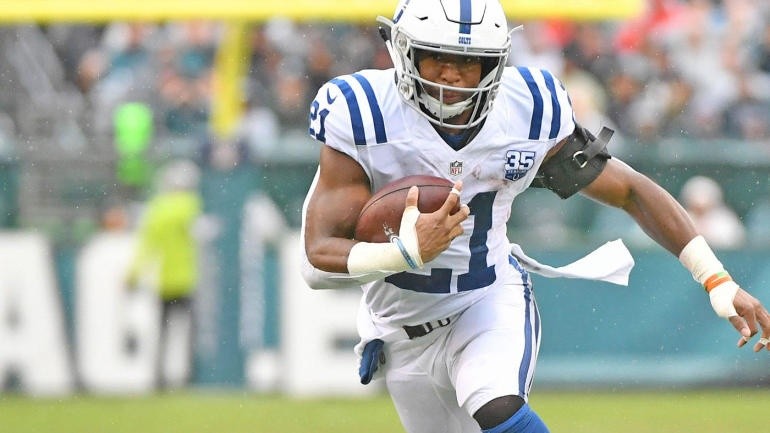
[402,314,450,339]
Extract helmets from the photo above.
[390,0,511,129]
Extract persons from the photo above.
[299,0,770,433]
[1,0,770,161]
[128,182,197,390]
[680,177,745,249]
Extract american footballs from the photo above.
[353,174,460,243]
